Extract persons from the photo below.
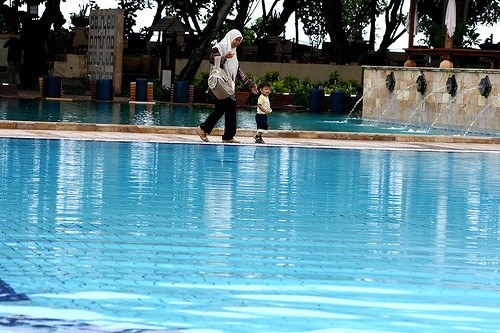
[3,31,24,84]
[195,28,259,143]
[252,82,273,144]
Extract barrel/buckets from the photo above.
[308,89,324,112]
[96,78,113,100]
[41,76,61,98]
[173,81,189,102]
[329,90,346,113]
[135,79,147,102]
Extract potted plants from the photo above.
[235,71,254,105]
[268,80,296,106]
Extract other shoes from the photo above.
[253,134,265,143]
[223,138,240,143]
[195,125,209,141]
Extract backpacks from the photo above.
[205,56,234,100]
